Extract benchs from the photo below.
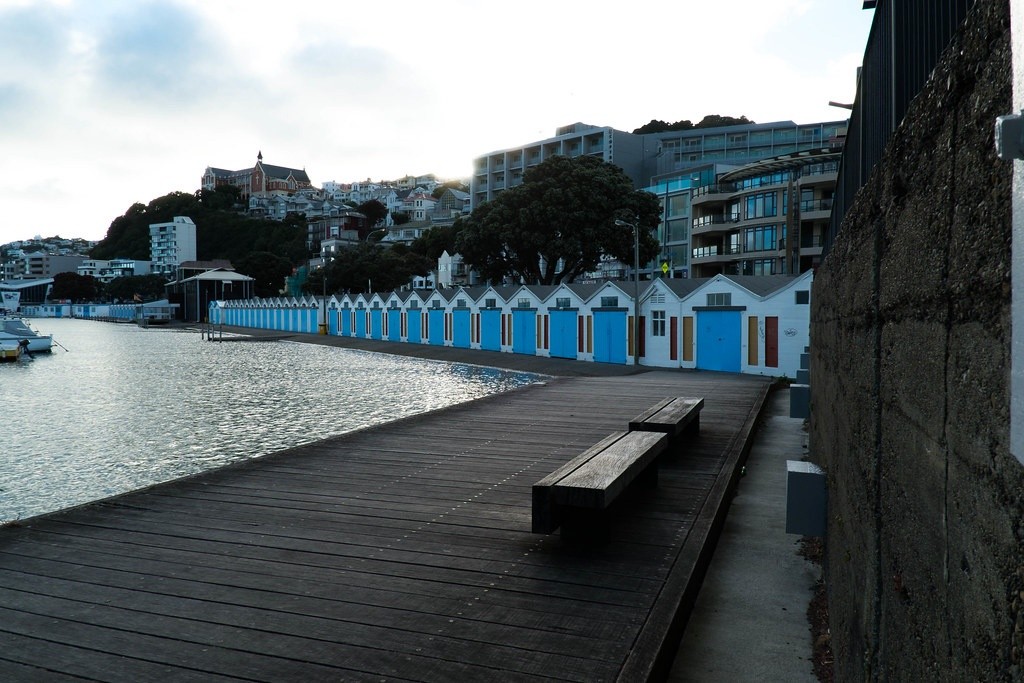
[530,431,664,541]
[630,396,704,456]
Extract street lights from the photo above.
[615,217,640,365]
[365,228,386,244]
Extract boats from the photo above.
[0,307,57,353]
[0,338,36,359]
